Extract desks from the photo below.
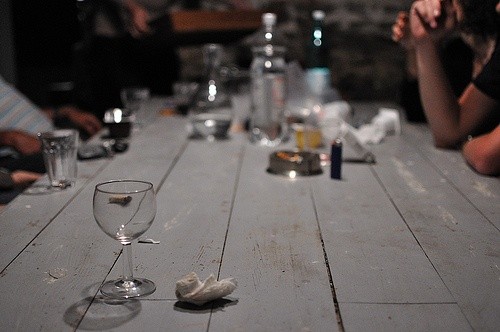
[0,95,500,332]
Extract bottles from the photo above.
[249,12,288,146]
[187,42,232,141]
[302,10,332,97]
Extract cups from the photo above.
[37,129,79,192]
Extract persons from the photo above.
[0,0,254,206]
[390,0,500,176]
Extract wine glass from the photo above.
[93,181,157,299]
